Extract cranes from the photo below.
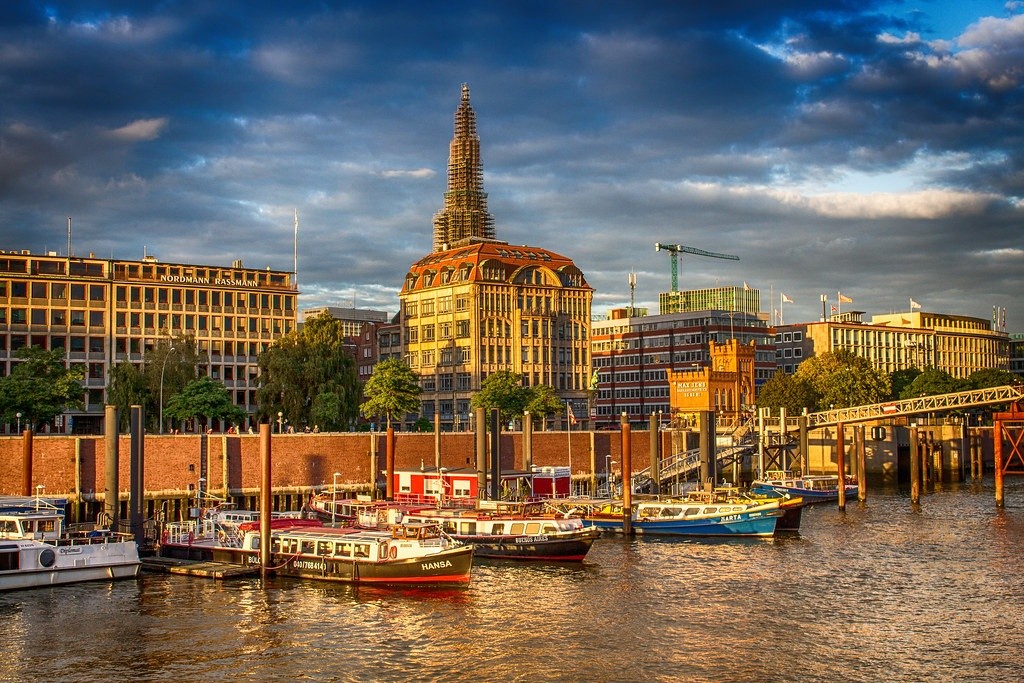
[655,243,740,312]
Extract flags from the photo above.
[569,406,577,425]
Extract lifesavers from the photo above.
[218,530,226,543]
[189,532,193,541]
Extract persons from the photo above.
[691,413,696,426]
[145,425,320,435]
[684,413,689,427]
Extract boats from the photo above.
[308,485,806,560]
[159,509,477,585]
[751,472,859,509]
[0,485,142,591]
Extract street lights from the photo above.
[965,413,970,428]
[160,347,176,434]
[278,412,283,434]
[332,472,342,528]
